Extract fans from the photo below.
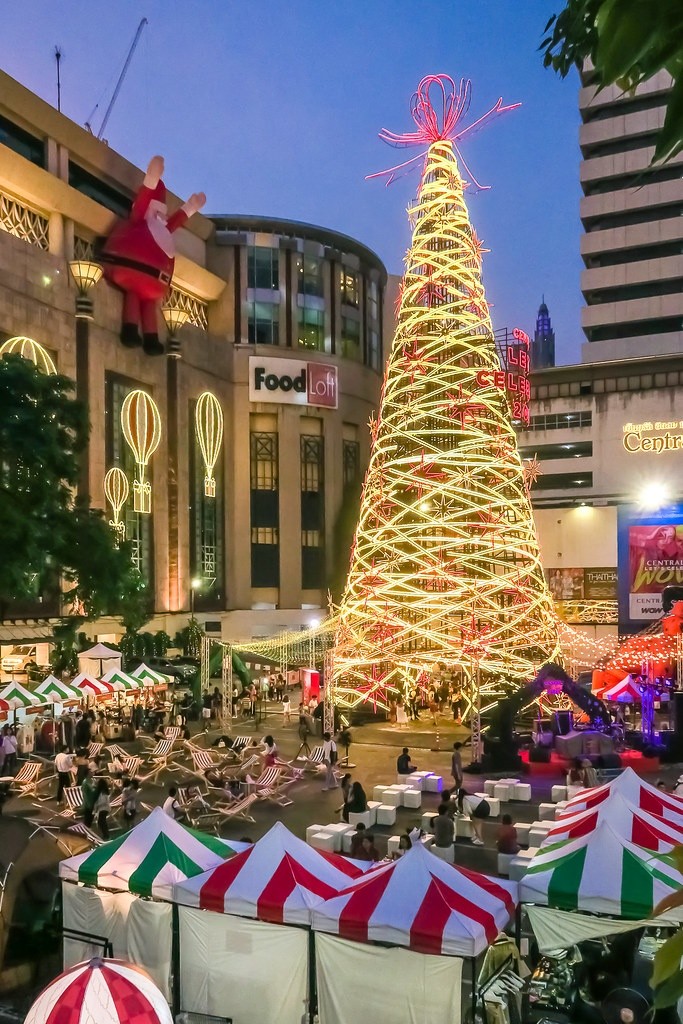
[336,731,357,768]
[294,725,312,761]
[601,986,653,1024]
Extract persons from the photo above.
[434,789,458,848]
[673,775,683,795]
[388,834,412,860]
[448,742,462,792]
[657,782,665,790]
[625,704,630,721]
[0,674,366,838]
[388,669,463,730]
[458,788,490,845]
[352,822,369,859]
[496,814,520,854]
[397,748,417,774]
[363,836,379,861]
[577,759,600,787]
[103,156,206,354]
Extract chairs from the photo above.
[0,726,327,851]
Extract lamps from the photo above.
[655,676,665,687]
[638,685,649,693]
[665,678,681,689]
[655,689,666,696]
[630,673,638,682]
[639,674,650,684]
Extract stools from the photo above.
[307,768,586,881]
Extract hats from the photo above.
[354,823,365,830]
[677,775,682,784]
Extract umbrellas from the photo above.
[23,955,174,1024]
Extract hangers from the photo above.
[470,968,526,1008]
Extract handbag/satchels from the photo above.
[218,739,226,747]
[329,741,338,765]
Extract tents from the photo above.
[77,643,122,678]
[0,663,174,753]
[59,806,520,1024]
[521,766,683,920]
[592,674,670,730]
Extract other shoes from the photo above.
[334,808,342,814]
[262,699,270,702]
[321,785,329,792]
[56,800,63,806]
[330,784,339,788]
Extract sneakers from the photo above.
[471,832,484,845]
